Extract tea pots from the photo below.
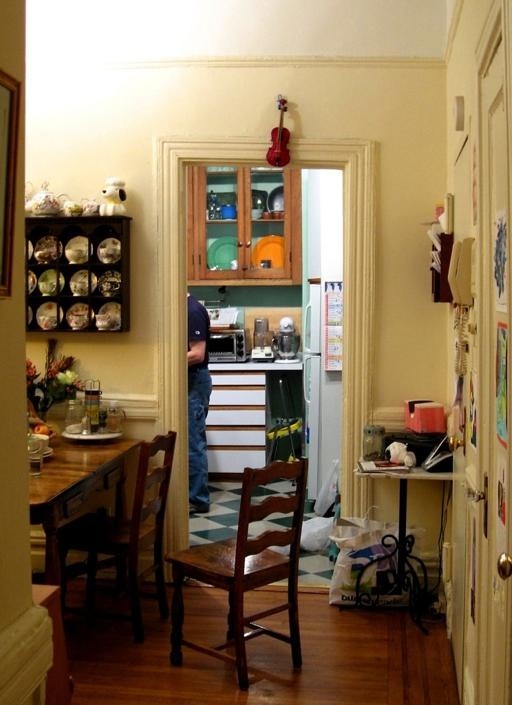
[23,182,71,218]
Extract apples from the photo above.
[34,425,50,436]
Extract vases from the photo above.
[35,409,48,422]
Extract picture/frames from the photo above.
[0,67,23,301]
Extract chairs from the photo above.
[54,428,177,643]
[167,458,313,692]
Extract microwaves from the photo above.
[209,327,251,362]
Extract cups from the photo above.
[67,314,86,331]
[220,204,236,219]
[65,247,87,264]
[34,249,56,264]
[38,315,58,331]
[98,247,121,264]
[26,438,44,477]
[260,259,272,270]
[95,312,112,331]
[38,281,57,297]
[69,280,88,297]
[263,211,287,220]
[101,280,120,297]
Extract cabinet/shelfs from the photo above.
[184,161,302,284]
[202,360,304,481]
[24,215,133,333]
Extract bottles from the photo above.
[207,191,217,220]
[374,425,386,461]
[65,400,84,434]
[215,207,221,219]
[107,399,126,432]
[81,413,91,435]
[362,425,377,460]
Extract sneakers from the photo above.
[189,502,209,513]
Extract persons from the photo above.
[186,287,213,516]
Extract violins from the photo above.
[267,99,290,166]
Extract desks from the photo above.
[356,463,457,601]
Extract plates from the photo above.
[205,236,239,270]
[37,268,65,296]
[34,234,63,263]
[97,269,121,297]
[98,302,121,331]
[42,447,53,455]
[266,185,284,214]
[65,303,94,330]
[28,449,53,461]
[96,237,122,264]
[28,305,33,325]
[27,269,38,295]
[69,269,97,296]
[35,301,64,331]
[251,234,285,270]
[28,240,33,260]
[65,236,94,264]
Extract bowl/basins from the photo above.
[26,434,50,453]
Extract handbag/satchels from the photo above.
[329,505,427,608]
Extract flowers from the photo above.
[24,335,84,409]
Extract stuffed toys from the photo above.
[99,176,130,216]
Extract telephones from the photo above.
[447,237,475,375]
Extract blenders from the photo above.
[271,318,301,364]
[252,319,273,362]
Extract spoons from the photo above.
[251,208,263,219]
